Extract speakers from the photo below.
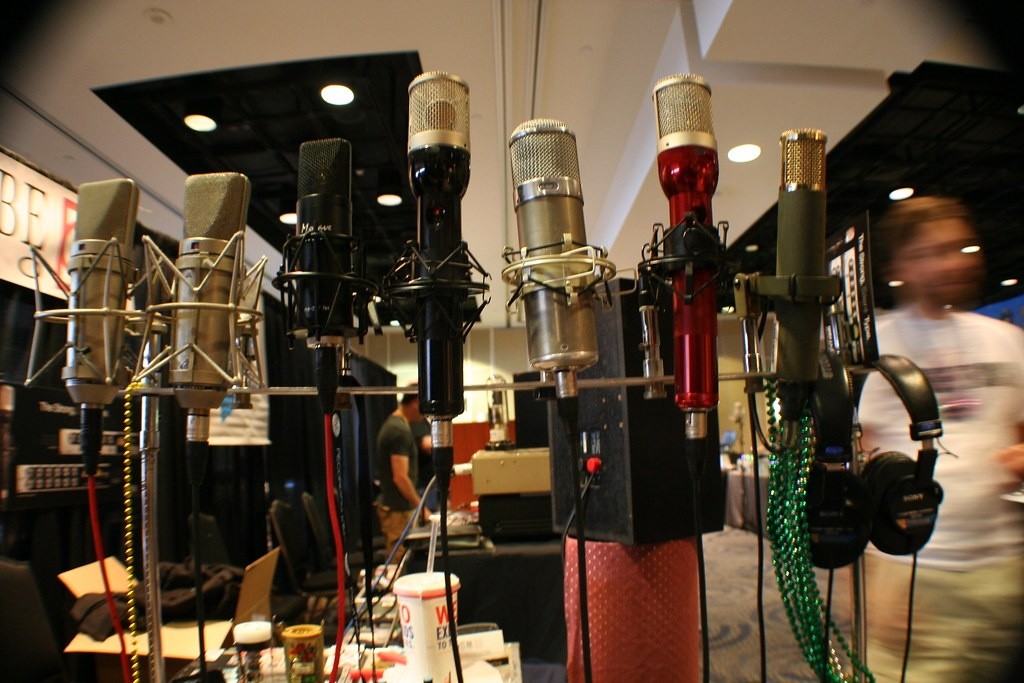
[544,279,726,546]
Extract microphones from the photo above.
[773,128,827,449]
[294,137,353,416]
[63,178,139,475]
[405,71,471,485]
[508,117,599,438]
[170,173,252,483]
[652,73,720,480]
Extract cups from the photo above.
[231,621,276,683]
[392,571,461,683]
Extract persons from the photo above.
[854,198,1024,681]
[373,383,438,564]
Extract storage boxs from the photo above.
[58,548,280,683]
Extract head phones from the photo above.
[802,354,944,570]
